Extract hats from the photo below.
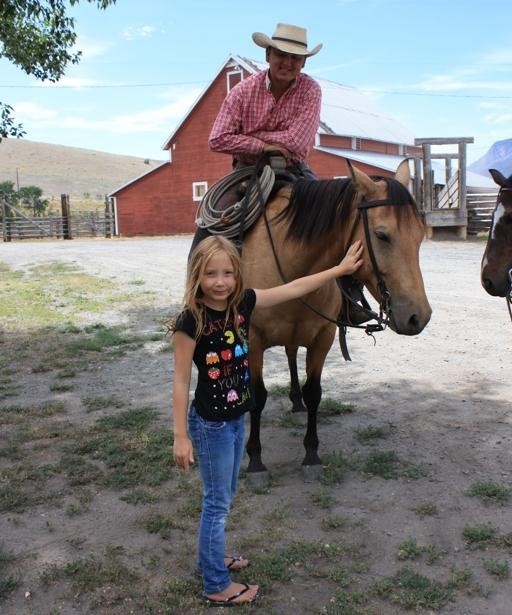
[252,22,325,59]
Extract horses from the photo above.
[480,168,512,322]
[187,158,433,494]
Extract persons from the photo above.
[172,234,364,607]
[207,22,377,325]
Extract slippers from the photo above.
[207,581,260,606]
[226,554,250,569]
